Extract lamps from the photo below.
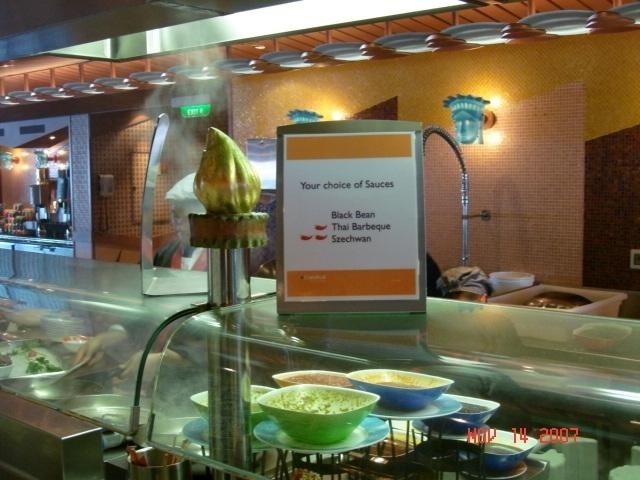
[442,92,496,147]
[286,108,322,125]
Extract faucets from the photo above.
[421,126,469,267]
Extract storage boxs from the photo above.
[486,282,629,343]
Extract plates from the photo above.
[461,460,529,479]
[369,395,463,419]
[252,414,390,454]
[182,417,273,450]
[1,2,639,108]
[489,268,535,296]
[41,314,83,343]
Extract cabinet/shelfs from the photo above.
[0,250,281,480]
[144,289,640,480]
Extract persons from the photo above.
[435,266,534,367]
[70,171,209,408]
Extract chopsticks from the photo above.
[124,438,192,465]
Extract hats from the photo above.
[436,266,493,295]
[165,172,205,210]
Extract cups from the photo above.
[124,447,189,479]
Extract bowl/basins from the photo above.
[467,428,539,474]
[345,369,455,409]
[410,419,490,440]
[192,385,275,422]
[422,393,500,433]
[571,323,632,352]
[0,362,14,379]
[61,334,93,351]
[258,382,382,444]
[271,369,353,389]
[352,431,425,460]
